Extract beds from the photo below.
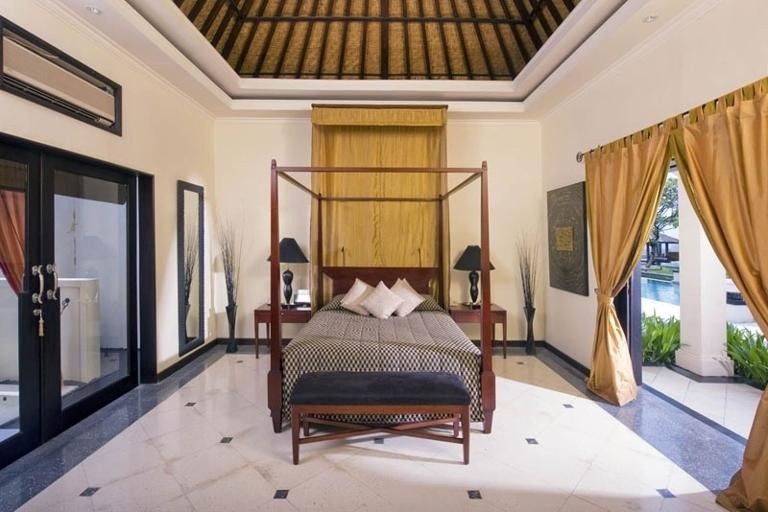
[267,266,496,422]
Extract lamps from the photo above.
[453,246,496,308]
[266,238,310,309]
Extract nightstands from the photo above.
[254,304,310,359]
[451,304,506,358]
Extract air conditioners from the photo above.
[2,37,115,126]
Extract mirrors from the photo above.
[177,180,204,357]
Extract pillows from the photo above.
[318,277,445,320]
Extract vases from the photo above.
[225,306,238,353]
[524,307,536,354]
[184,305,190,344]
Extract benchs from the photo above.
[290,372,470,464]
